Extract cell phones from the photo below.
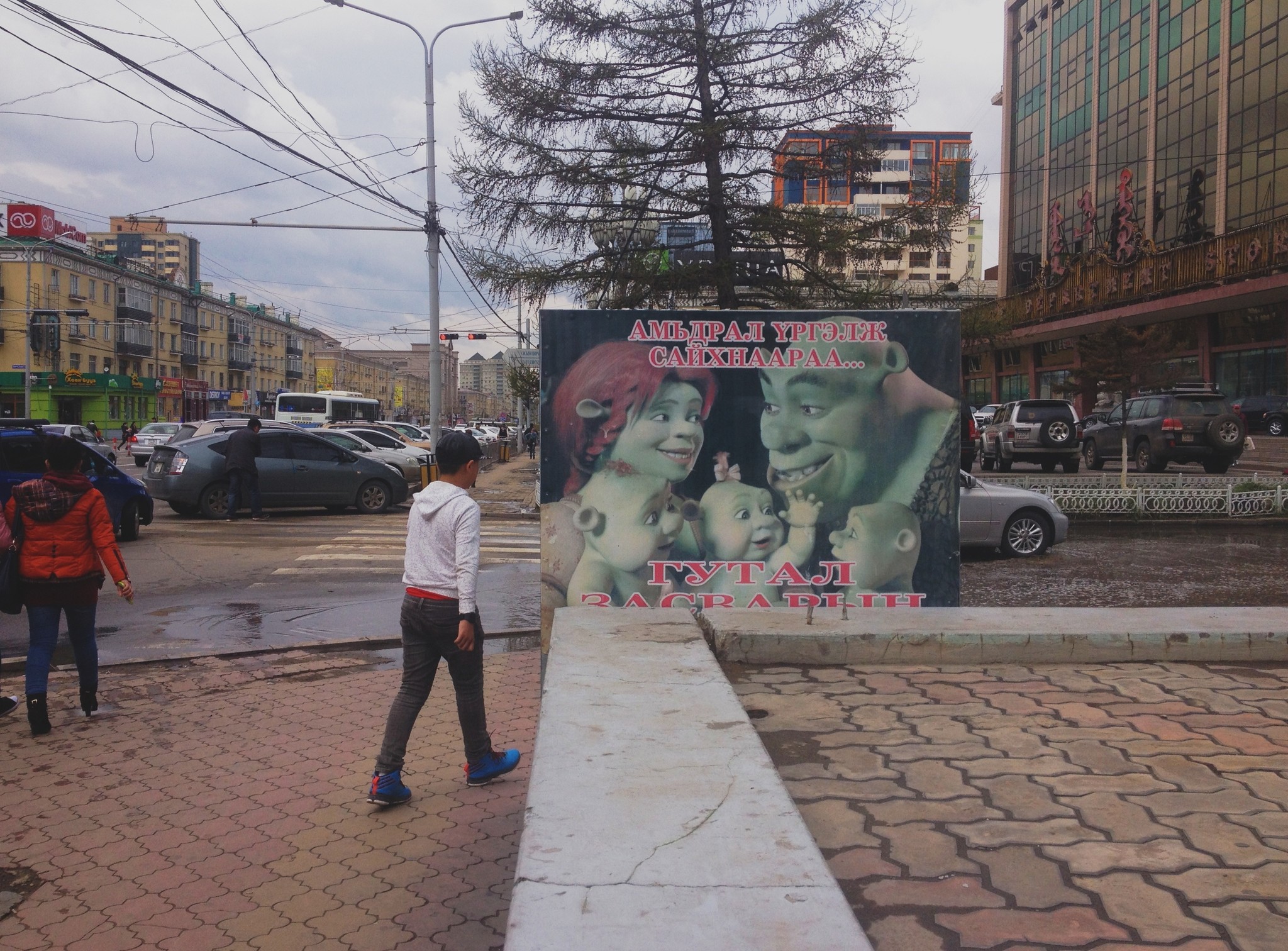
[118,581,133,605]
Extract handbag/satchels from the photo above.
[0,502,24,614]
[525,444,530,452]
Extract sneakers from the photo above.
[0,695,19,718]
[464,749,521,786]
[366,769,412,807]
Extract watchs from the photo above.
[460,612,476,623]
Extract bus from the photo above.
[275,390,385,429]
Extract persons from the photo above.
[0,434,134,734]
[86,417,158,451]
[225,418,270,522]
[476,422,539,460]
[364,430,520,805]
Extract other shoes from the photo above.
[252,512,270,520]
[533,456,535,459]
[530,456,532,459]
[117,447,120,451]
[125,447,128,450]
[226,517,238,522]
[472,486,476,488]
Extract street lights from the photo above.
[0,231,73,418]
[380,357,408,422]
[324,339,361,391]
[325,0,523,463]
[225,305,281,414]
[446,382,463,429]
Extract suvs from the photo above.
[165,411,314,445]
[1082,382,1245,473]
[979,399,1083,473]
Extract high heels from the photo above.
[79,682,98,717]
[27,693,52,737]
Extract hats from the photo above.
[437,430,493,461]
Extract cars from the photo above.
[1231,396,1288,436]
[0,418,154,541]
[141,429,409,520]
[130,423,185,467]
[303,420,526,483]
[22,424,116,466]
[960,394,1003,473]
[960,470,1068,556]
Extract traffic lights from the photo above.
[468,333,487,340]
[440,333,459,340]
